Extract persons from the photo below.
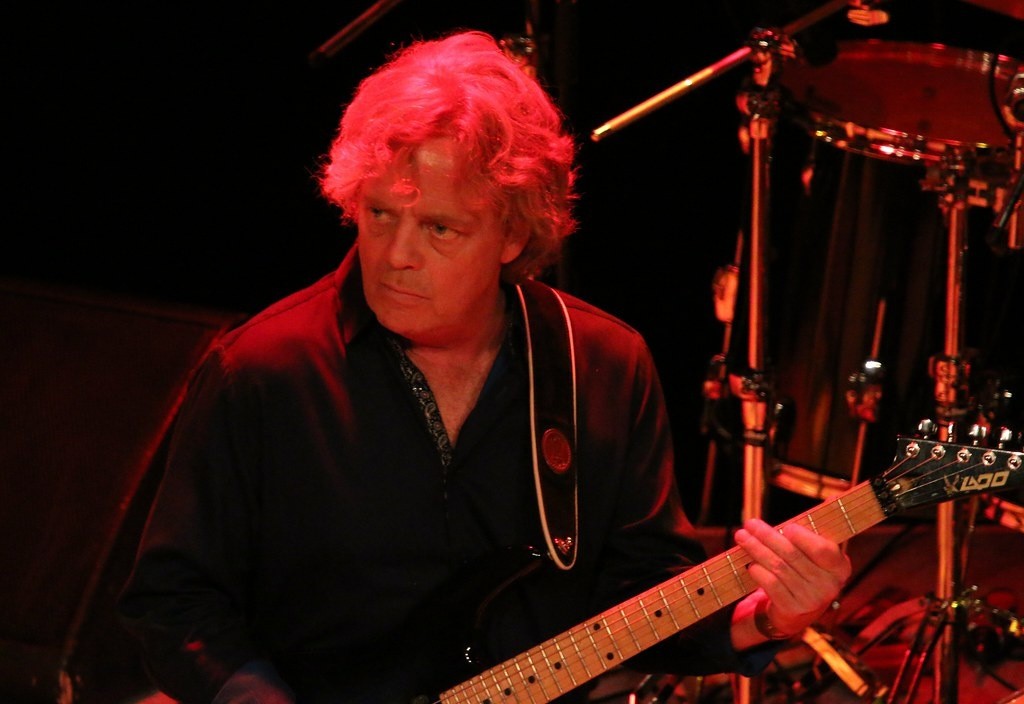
[116,31,853,704]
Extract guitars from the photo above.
[420,419,1024,704]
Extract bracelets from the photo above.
[753,594,807,642]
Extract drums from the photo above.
[765,38,1024,504]
